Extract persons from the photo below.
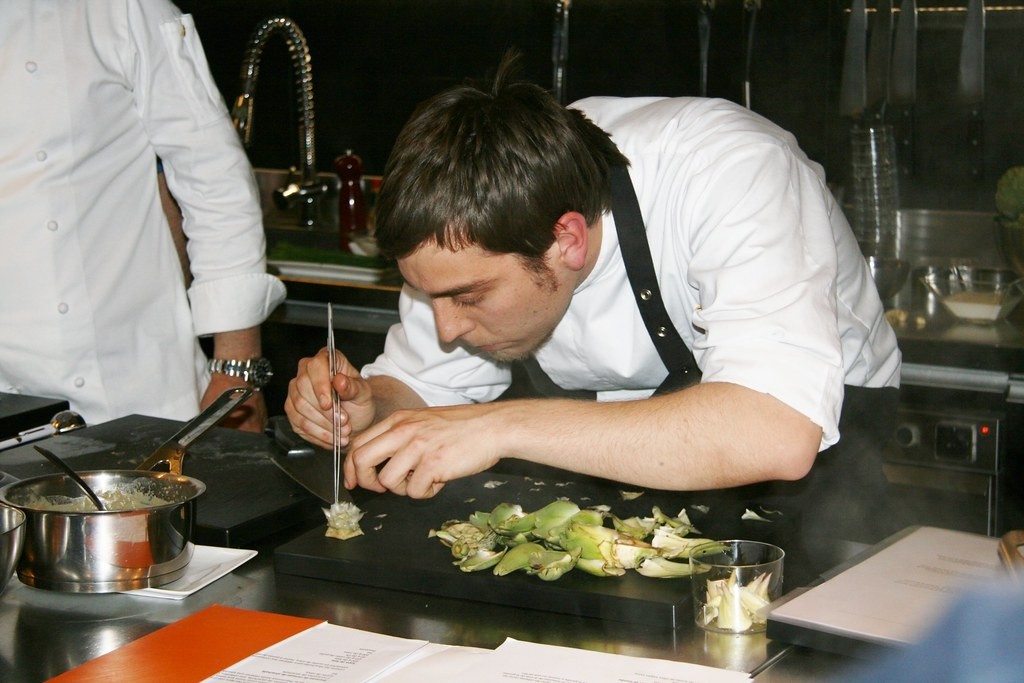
[0,2,287,438]
[282,82,908,500]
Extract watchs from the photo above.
[206,354,274,386]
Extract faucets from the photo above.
[232,15,332,229]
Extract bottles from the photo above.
[336,148,366,254]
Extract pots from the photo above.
[0,386,255,588]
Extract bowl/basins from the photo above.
[864,255,1024,323]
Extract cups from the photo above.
[689,539,785,634]
[852,125,897,255]
